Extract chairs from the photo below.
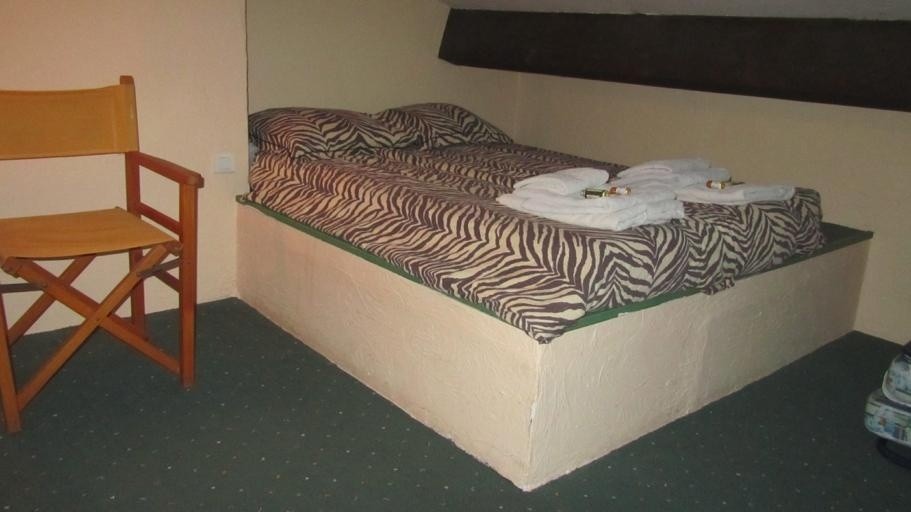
[0,71,205,437]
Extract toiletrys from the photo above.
[610,186,631,195]
[580,186,608,199]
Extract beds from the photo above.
[238,140,872,495]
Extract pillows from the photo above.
[248,101,514,159]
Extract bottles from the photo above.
[581,185,631,198]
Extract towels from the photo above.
[493,167,685,232]
[617,157,795,206]
